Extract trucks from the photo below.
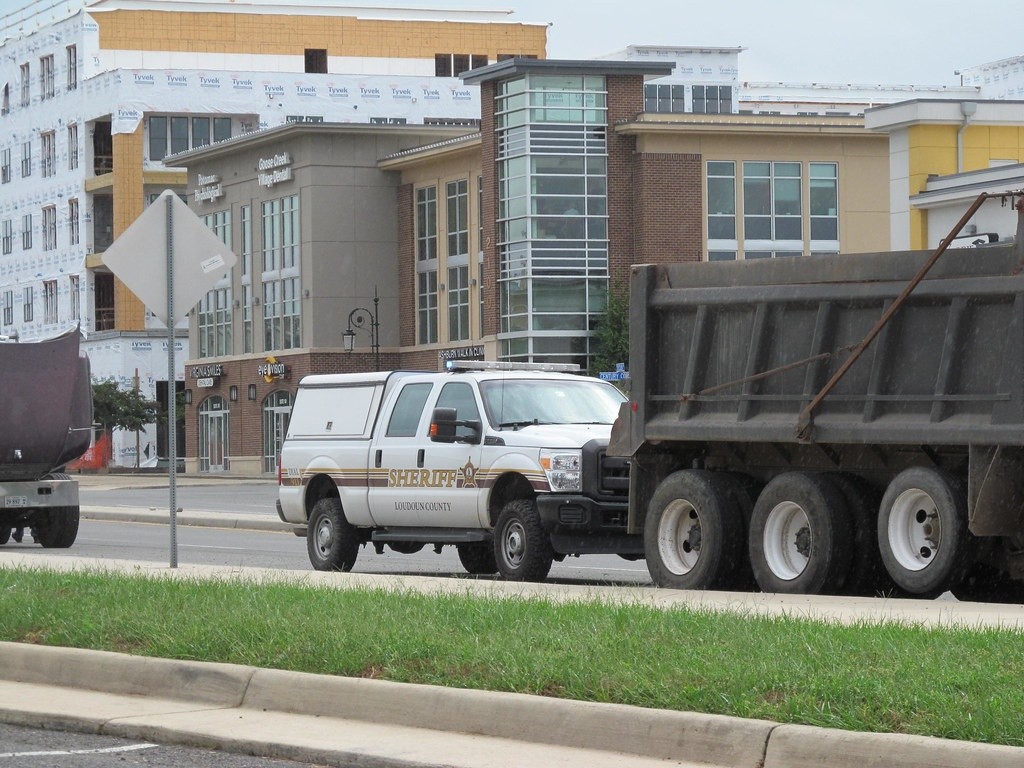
[0,337,98,547]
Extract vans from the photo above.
[274,358,628,581]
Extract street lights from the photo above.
[341,305,379,371]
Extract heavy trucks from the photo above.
[606,190,1023,605]
